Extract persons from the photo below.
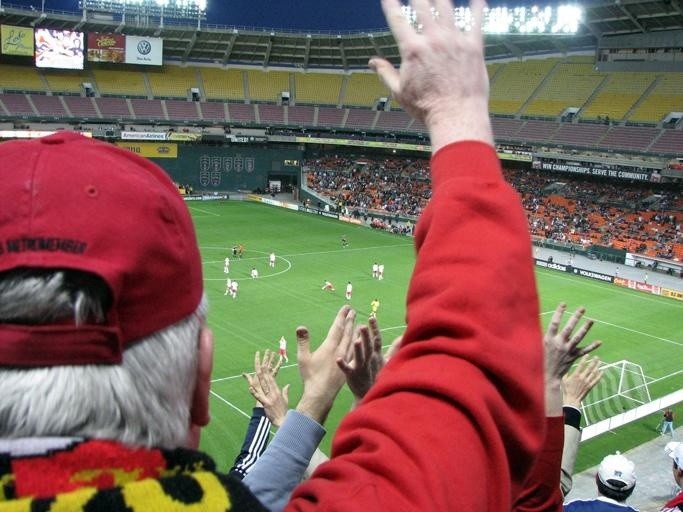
[179,181,194,196]
[223,277,232,295]
[250,268,257,280]
[269,252,275,268]
[231,279,238,298]
[0,3,550,509]
[295,132,682,290]
[232,245,237,257]
[10,123,293,133]
[223,257,229,273]
[238,243,243,260]
[217,290,682,511]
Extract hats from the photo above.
[598,454,637,491]
[664,441,683,470]
[0,130,204,371]
